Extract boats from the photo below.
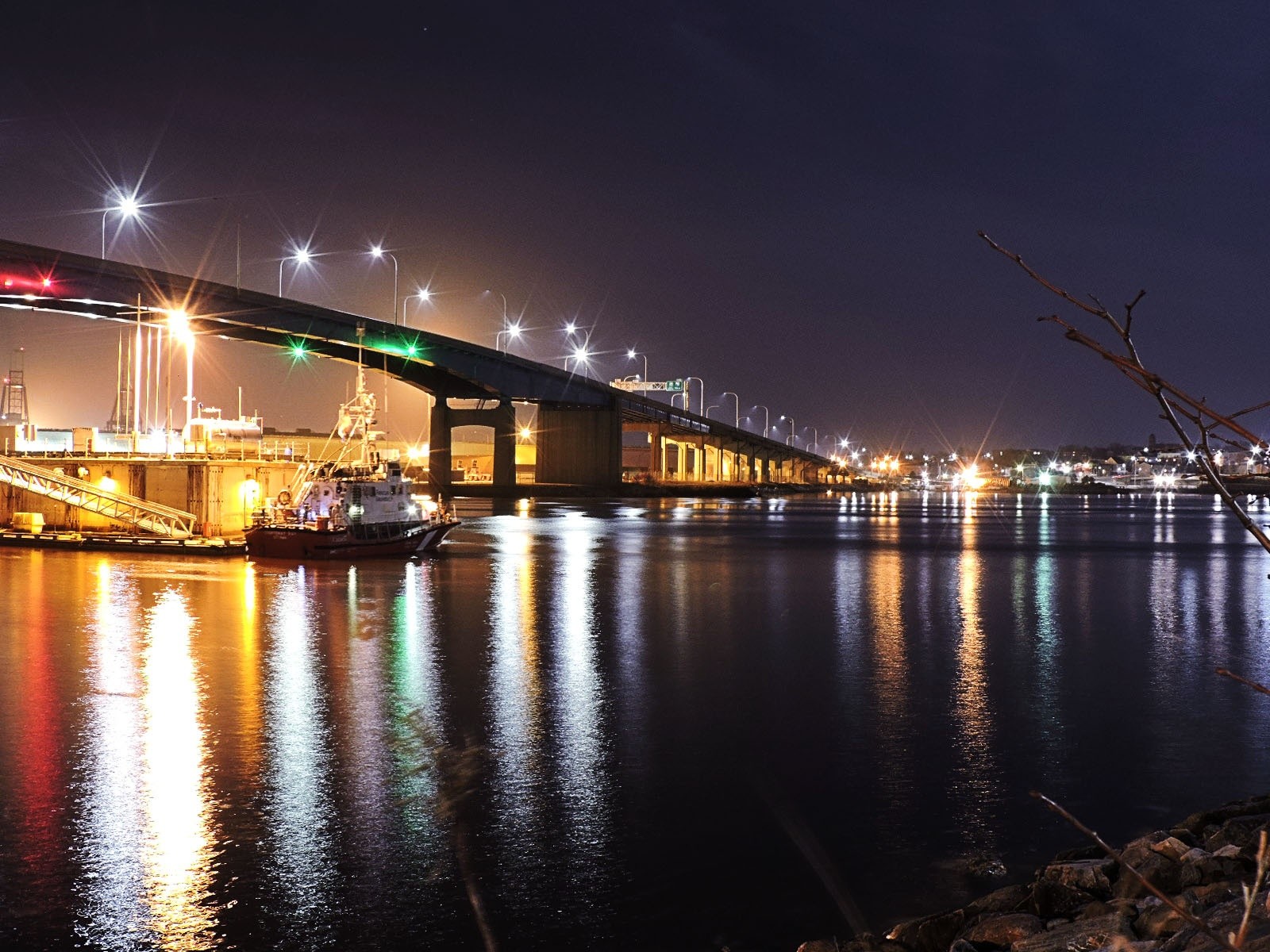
[244,366,463,559]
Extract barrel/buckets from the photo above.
[317,516,329,530]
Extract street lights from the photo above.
[101,206,134,259]
[755,405,768,440]
[279,256,307,299]
[624,374,639,383]
[825,435,873,471]
[689,377,704,418]
[564,353,584,372]
[786,434,799,447]
[403,293,428,327]
[723,392,738,430]
[372,248,398,327]
[496,329,519,352]
[706,405,720,419]
[568,327,589,382]
[781,416,795,449]
[630,352,647,400]
[805,426,818,454]
[763,426,776,439]
[486,288,507,357]
[806,443,819,453]
[671,393,686,408]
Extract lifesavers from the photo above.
[277,491,291,506]
